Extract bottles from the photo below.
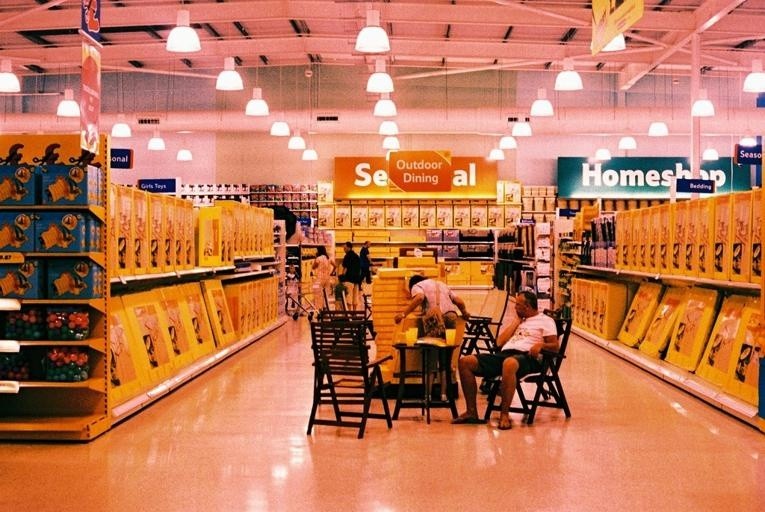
[580,240,592,265]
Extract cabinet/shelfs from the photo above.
[319,195,556,260]
[554,208,764,435]
[163,192,318,267]
[0,133,285,443]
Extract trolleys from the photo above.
[283,275,335,321]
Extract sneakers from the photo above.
[452,416,477,424]
[498,419,512,429]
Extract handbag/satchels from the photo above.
[421,305,446,337]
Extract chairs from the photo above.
[305,316,394,438]
[484,309,572,424]
[459,289,508,358]
[313,307,370,403]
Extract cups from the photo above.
[405,326,418,347]
[557,198,673,212]
[445,328,457,346]
[521,184,556,225]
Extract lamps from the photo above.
[354,0,399,160]
[485,34,765,161]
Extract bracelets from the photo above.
[402,312,406,318]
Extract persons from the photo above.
[358,240,374,291]
[311,246,337,320]
[286,262,300,317]
[333,274,349,316]
[450,290,560,430]
[340,241,362,316]
[394,274,471,401]
